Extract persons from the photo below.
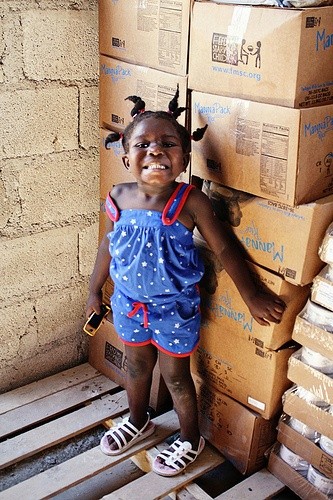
[85,85,286,476]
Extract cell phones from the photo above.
[84,303,111,336]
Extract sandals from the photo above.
[100,412,155,456]
[152,436,205,477]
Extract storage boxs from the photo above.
[87,0,333,500]
